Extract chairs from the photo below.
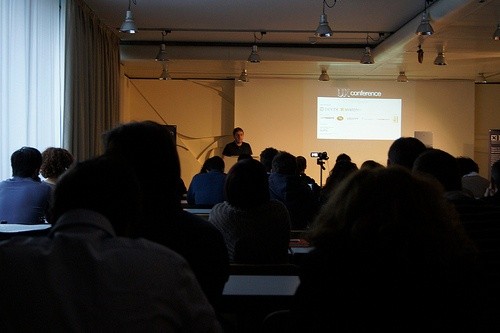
[217,264,303,333]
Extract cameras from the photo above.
[310,152,329,160]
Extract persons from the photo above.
[221,127,253,158]
[0,120,500,333]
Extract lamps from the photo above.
[153,31,172,82]
[319,70,330,82]
[313,0,332,38]
[246,32,262,64]
[358,34,375,64]
[492,24,500,40]
[431,51,448,65]
[237,68,249,82]
[415,0,434,35]
[117,0,140,34]
[397,71,409,83]
